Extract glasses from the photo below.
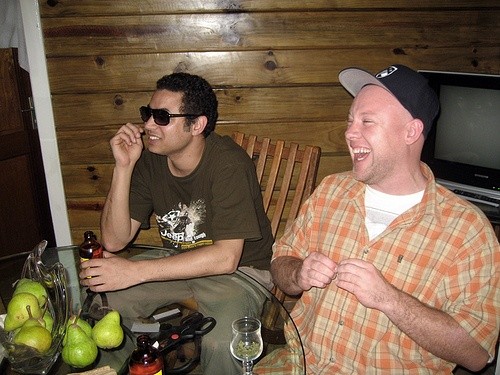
[140,104,201,127]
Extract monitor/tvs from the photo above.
[416,71,500,197]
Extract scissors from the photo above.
[157,312,216,353]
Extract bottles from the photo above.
[78,230,103,288]
[128,335,165,375]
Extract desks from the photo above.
[0,248,306,375]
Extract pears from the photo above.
[3,278,53,355]
[60,306,123,368]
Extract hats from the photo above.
[338,63,441,139]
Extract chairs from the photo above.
[231,132,321,354]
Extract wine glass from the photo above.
[230,317,263,375]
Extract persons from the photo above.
[251,64,500,375]
[79,72,275,375]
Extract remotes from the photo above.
[451,189,500,207]
